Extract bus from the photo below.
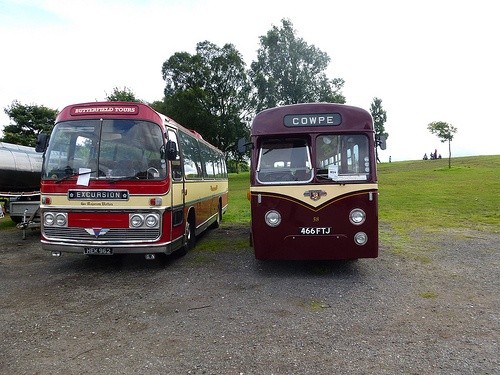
[34,101,229,261]
[237,101,386,263]
